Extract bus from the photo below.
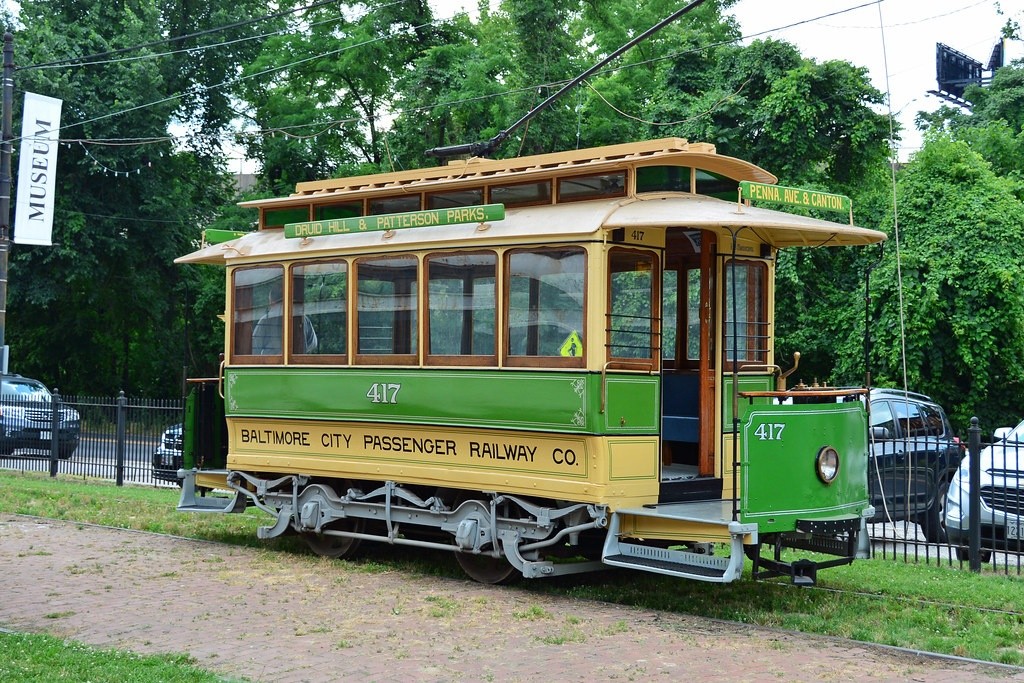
[174,137,888,585]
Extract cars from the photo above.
[151,423,214,493]
[944,420,1024,563]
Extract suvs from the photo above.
[772,386,969,544]
[0,373,81,458]
